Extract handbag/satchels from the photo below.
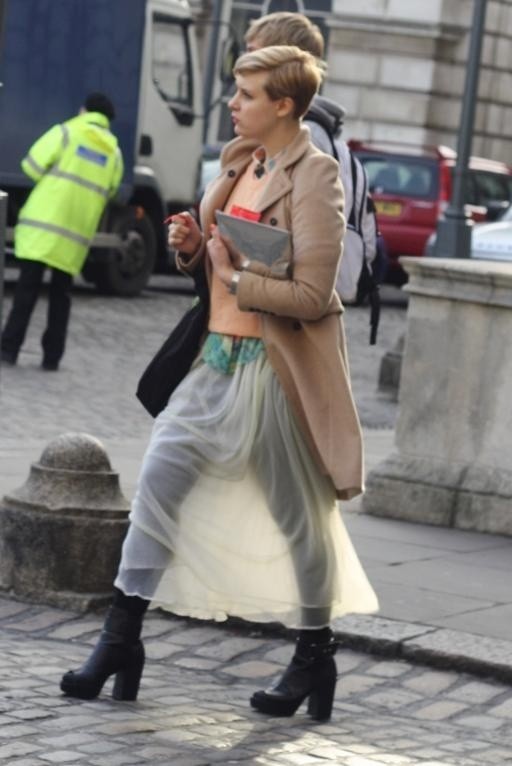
[136,301,209,418]
[301,112,391,307]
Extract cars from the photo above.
[346,137,512,288]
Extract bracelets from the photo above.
[228,271,241,295]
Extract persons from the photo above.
[0,92,125,371]
[60,45,380,721]
[243,12,380,305]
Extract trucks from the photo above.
[1,0,241,299]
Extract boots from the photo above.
[61,604,144,701]
[251,634,337,719]
